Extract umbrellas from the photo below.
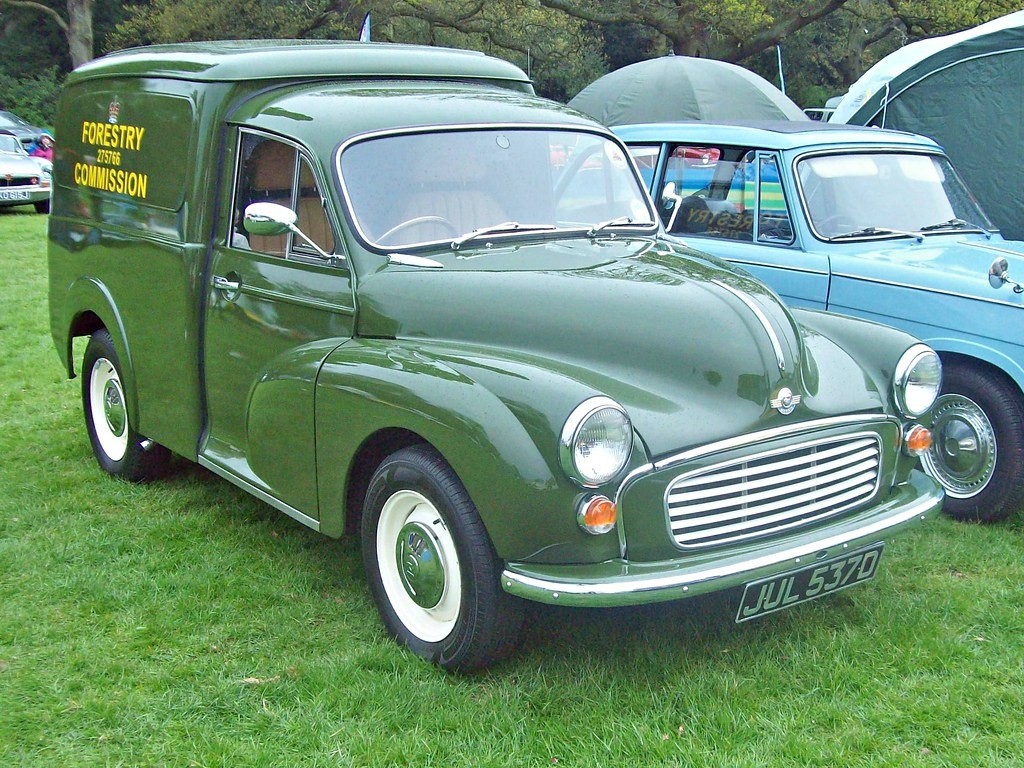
[567,49,812,127]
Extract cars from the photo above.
[546,121,1024,520]
[0,134,55,214]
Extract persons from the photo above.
[28,129,56,162]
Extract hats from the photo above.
[41,133,56,142]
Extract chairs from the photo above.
[245,140,335,256]
[663,194,714,236]
[398,139,511,242]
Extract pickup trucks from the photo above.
[49,40,947,673]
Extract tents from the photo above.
[800,9,1024,241]
